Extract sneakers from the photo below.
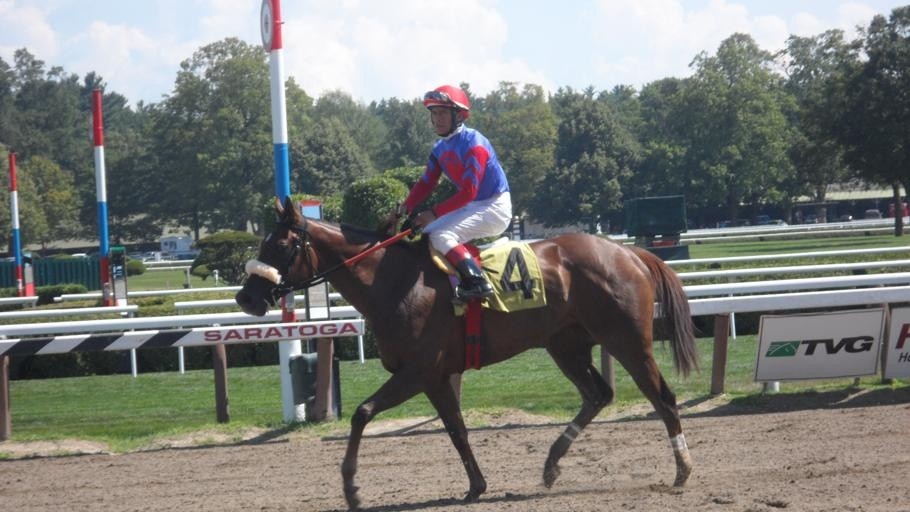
[455,274,496,300]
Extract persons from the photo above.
[384,83,513,305]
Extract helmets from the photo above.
[423,84,469,113]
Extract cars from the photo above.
[0,234,201,265]
[713,203,908,227]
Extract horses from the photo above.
[233,193,702,511]
